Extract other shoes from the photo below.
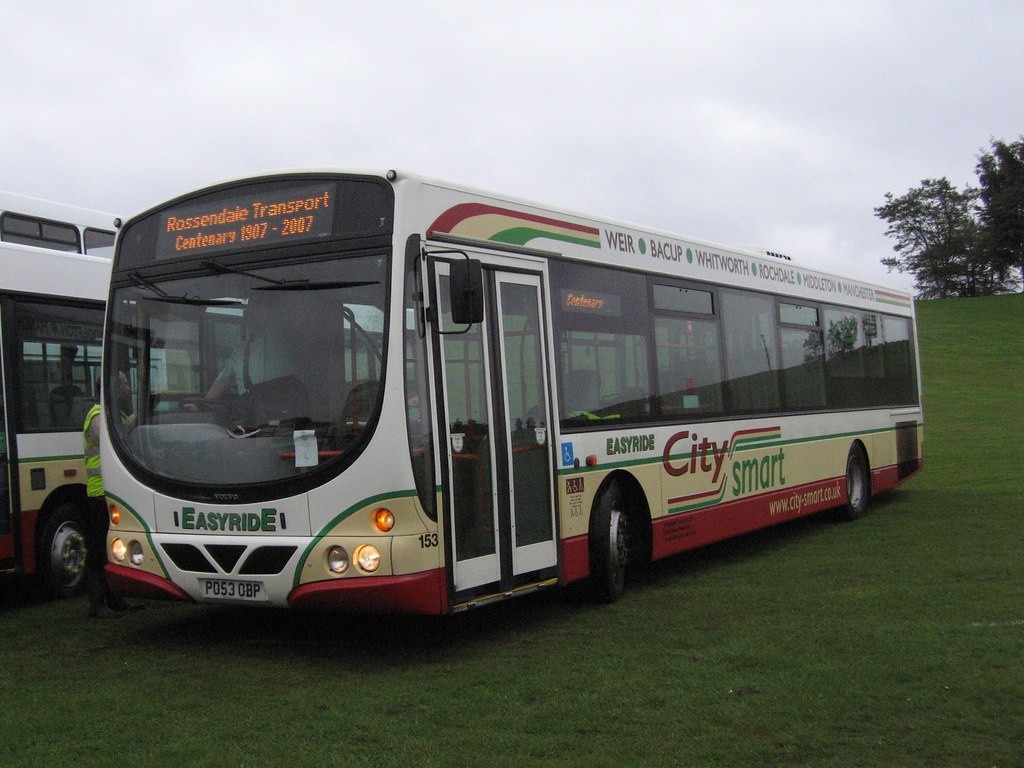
[90,607,122,619]
[107,598,146,611]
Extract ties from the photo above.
[243,338,253,389]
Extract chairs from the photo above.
[47,384,92,431]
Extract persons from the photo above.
[83,371,162,617]
[180,295,304,414]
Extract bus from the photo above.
[0,194,423,601]
[95,166,925,617]
